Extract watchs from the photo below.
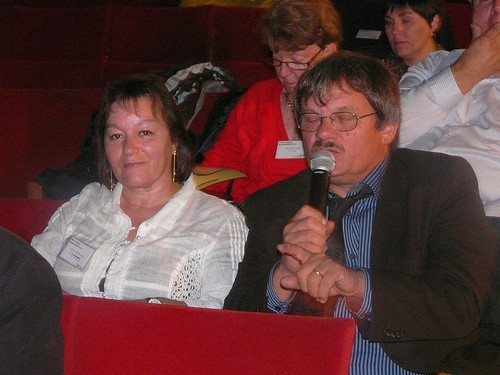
[145,298,162,304]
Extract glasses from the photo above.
[296,111,377,133]
[265,48,323,71]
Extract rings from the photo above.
[312,268,325,278]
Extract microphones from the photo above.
[308,150,337,220]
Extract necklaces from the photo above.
[285,93,295,110]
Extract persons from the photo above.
[396,0,500,375]
[380,0,446,81]
[188,0,342,204]
[29,71,249,310]
[0,224,65,375]
[228,48,494,375]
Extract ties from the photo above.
[289,183,373,317]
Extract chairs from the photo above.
[0,0,473,375]
